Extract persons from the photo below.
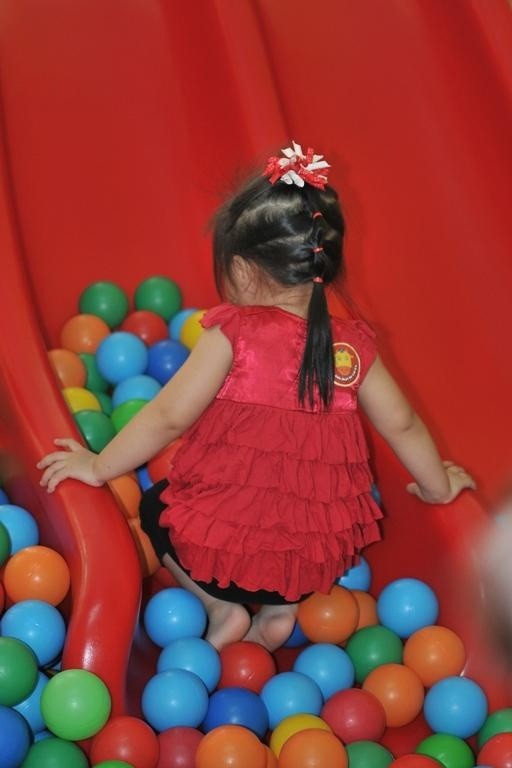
[36,139,477,649]
